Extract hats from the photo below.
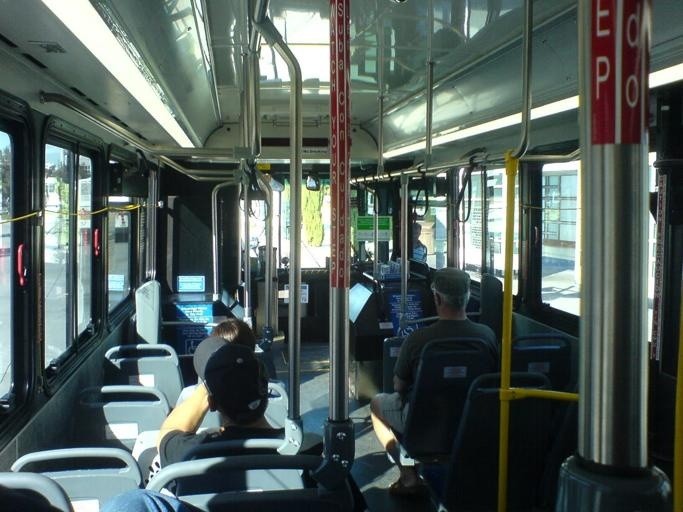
[430,267,470,296]
[193,336,266,415]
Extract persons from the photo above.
[156,333,324,469]
[412,220,429,261]
[367,265,500,503]
[173,319,285,408]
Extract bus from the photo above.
[76,177,131,241]
